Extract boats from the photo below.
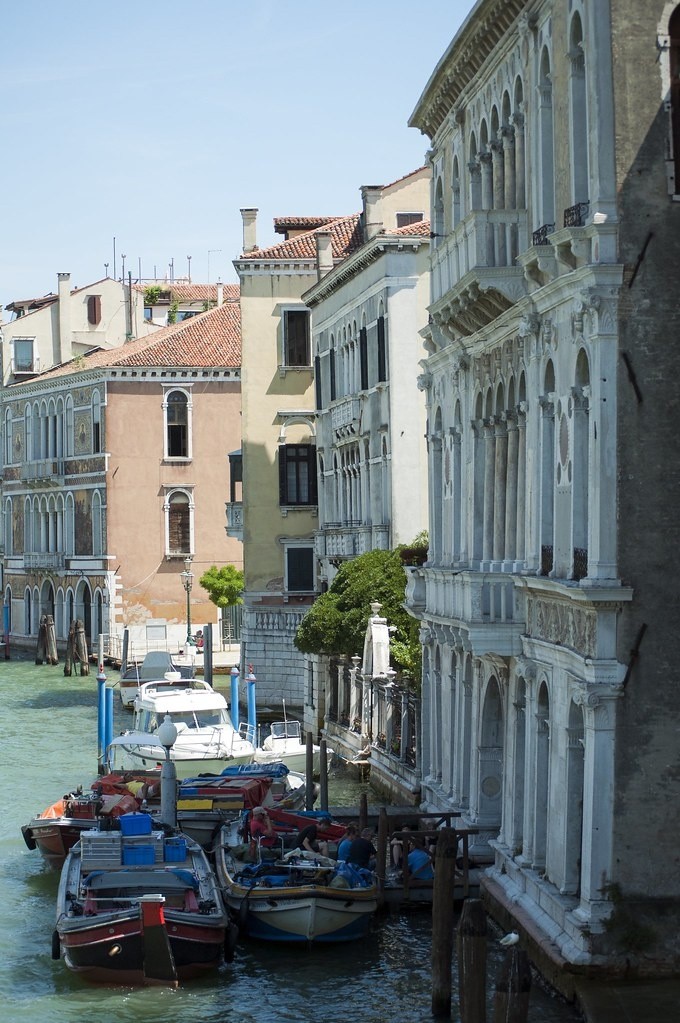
[21,650,381,989]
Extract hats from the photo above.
[253,807,267,815]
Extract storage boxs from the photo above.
[123,845,155,866]
[79,830,121,860]
[121,831,165,864]
[118,814,151,836]
[163,843,186,863]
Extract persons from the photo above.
[296,819,331,855]
[428,838,464,879]
[390,824,417,868]
[425,823,444,859]
[250,806,289,848]
[337,822,361,863]
[350,828,377,871]
[395,839,434,884]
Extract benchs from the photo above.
[93,770,161,800]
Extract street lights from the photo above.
[179,555,195,646]
